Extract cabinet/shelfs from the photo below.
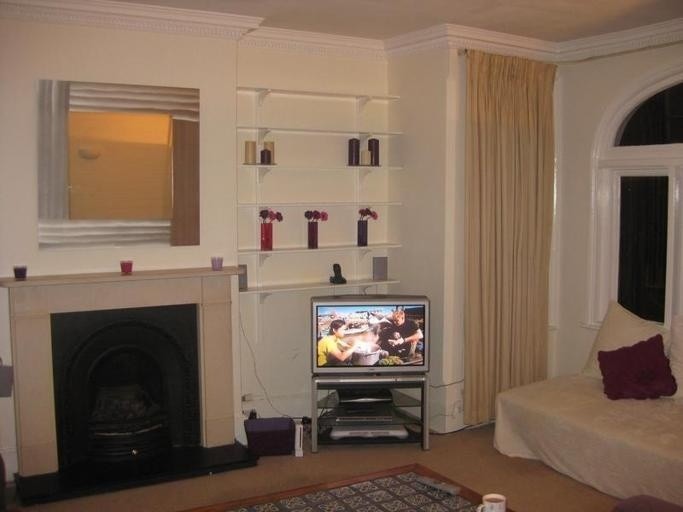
[310,370,430,455]
[234,83,410,297]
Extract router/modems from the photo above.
[294,422,305,458]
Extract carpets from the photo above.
[177,460,519,512]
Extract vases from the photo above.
[261,223,273,251]
[307,220,318,249]
[356,219,368,247]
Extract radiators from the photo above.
[356,207,378,221]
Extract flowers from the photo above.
[303,209,330,223]
[258,208,282,224]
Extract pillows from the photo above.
[582,297,667,387]
[667,313,681,398]
[596,330,678,400]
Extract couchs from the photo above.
[491,365,682,510]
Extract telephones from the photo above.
[330,264,346,284]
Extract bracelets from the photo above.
[404,338,406,344]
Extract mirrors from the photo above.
[34,75,202,252]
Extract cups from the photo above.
[259,150,270,165]
[244,141,256,165]
[13,265,26,280]
[237,264,247,290]
[119,260,131,276]
[211,256,223,270]
[475,492,507,511]
[262,140,274,165]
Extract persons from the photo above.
[380,310,424,357]
[317,320,354,367]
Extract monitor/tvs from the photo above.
[311,294,431,375]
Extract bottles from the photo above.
[348,139,359,166]
[367,138,379,166]
